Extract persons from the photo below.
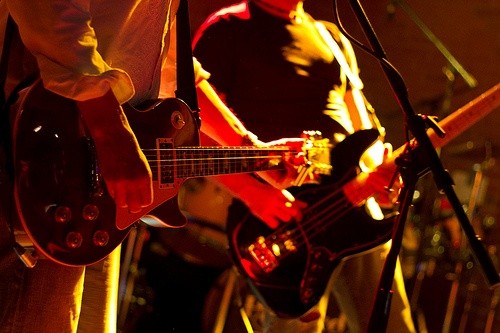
[188,1,417,333]
[0,0,323,333]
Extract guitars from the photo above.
[1,71,334,269]
[225,80,500,322]
